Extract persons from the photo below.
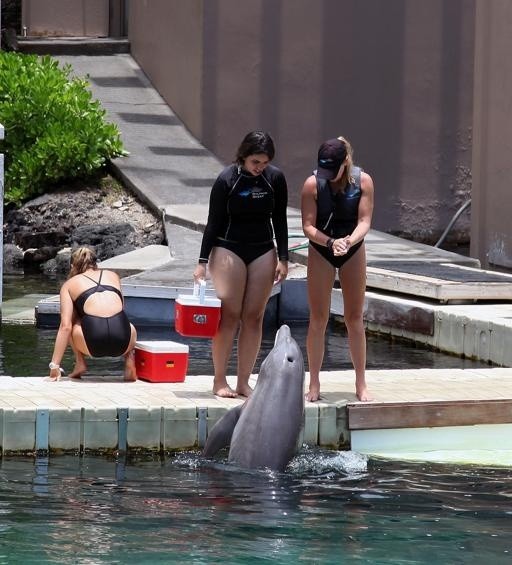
[48,246,140,382]
[300,135,379,403]
[192,129,290,398]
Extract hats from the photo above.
[317,138,347,180]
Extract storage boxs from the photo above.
[133,340,190,384]
[176,279,222,337]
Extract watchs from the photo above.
[49,362,59,369]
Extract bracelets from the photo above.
[327,237,334,247]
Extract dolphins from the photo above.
[202,323,311,474]
